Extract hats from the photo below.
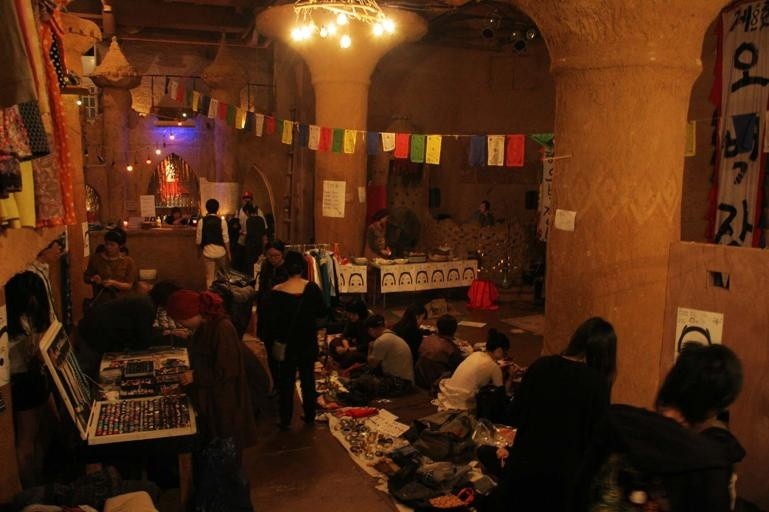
[242,191,253,201]
[36,224,65,250]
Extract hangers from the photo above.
[313,241,330,258]
[288,243,307,257]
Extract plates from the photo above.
[379,260,394,265]
[350,256,369,265]
[394,259,409,264]
[371,258,383,262]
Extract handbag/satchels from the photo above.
[270,286,307,362]
[82,274,111,315]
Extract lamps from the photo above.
[126,161,132,171]
[169,130,175,140]
[146,151,152,164]
[290,0,394,48]
[154,144,161,155]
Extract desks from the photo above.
[370,258,478,310]
[333,258,368,306]
[91,222,215,292]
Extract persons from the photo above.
[496,315,617,512]
[430,329,511,416]
[236,190,268,256]
[166,288,259,473]
[165,207,183,226]
[415,315,463,390]
[570,341,745,512]
[364,208,391,262]
[353,314,414,398]
[195,199,231,288]
[328,301,372,367]
[270,251,322,431]
[393,304,428,360]
[82,228,140,301]
[243,201,265,270]
[256,240,285,382]
[472,200,495,227]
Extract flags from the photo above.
[297,123,357,153]
[191,90,219,119]
[255,113,293,145]
[165,78,193,106]
[365,131,442,165]
[469,134,525,167]
[219,103,254,130]
